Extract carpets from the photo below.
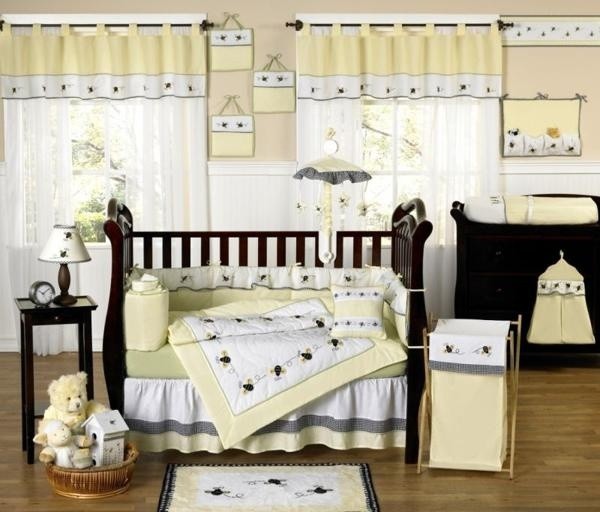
[156,463,379,512]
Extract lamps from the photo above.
[37,223,92,306]
[292,128,371,268]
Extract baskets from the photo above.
[44,443,139,498]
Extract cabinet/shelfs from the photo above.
[449,195,600,369]
[416,313,523,482]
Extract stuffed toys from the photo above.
[33,371,109,448]
[292,139,372,223]
[39,420,93,470]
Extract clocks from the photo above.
[28,280,56,306]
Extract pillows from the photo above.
[329,285,389,341]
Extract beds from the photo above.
[102,199,434,465]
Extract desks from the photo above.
[13,295,98,466]
